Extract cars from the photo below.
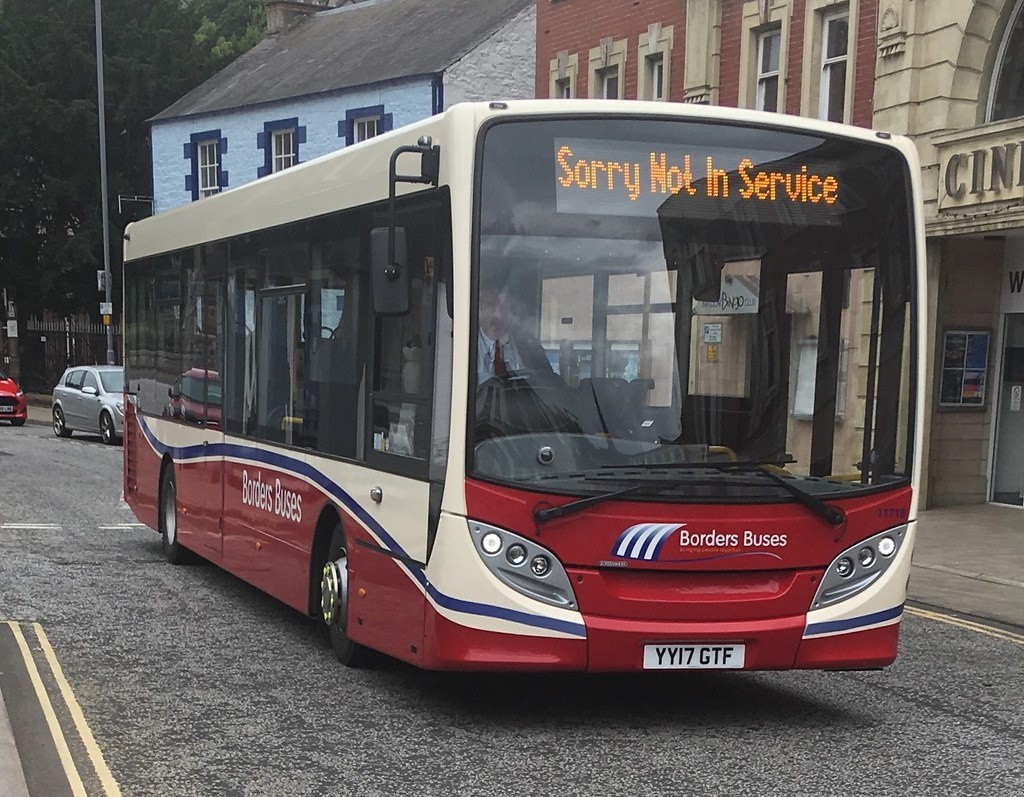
[51,365,123,444]
[0,369,28,427]
[166,369,222,429]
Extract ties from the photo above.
[494,339,508,380]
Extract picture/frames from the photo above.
[936,326,994,414]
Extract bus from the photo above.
[122,99,930,679]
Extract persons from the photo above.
[415,282,562,458]
